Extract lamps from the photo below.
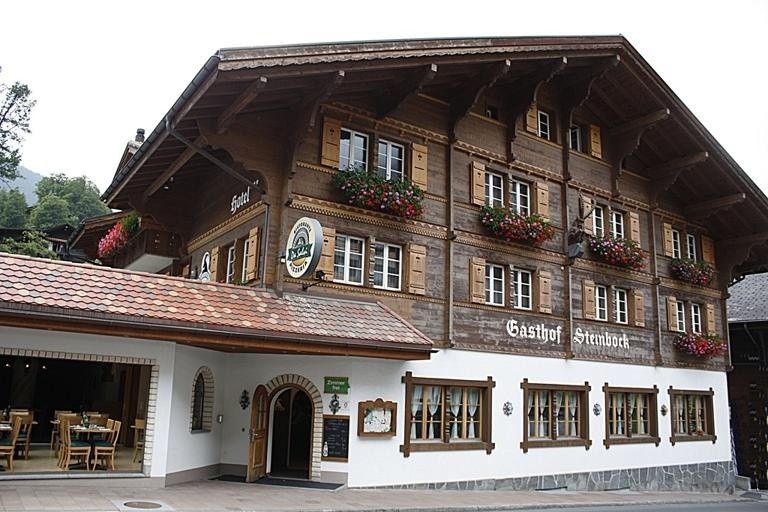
[239,390,251,411]
[327,392,341,412]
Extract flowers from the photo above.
[333,165,426,223]
[590,231,649,269]
[671,330,727,356]
[479,206,554,248]
[98,218,130,260]
[667,257,719,286]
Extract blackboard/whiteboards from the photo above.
[322,415,350,463]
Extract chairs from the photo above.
[0,409,146,470]
